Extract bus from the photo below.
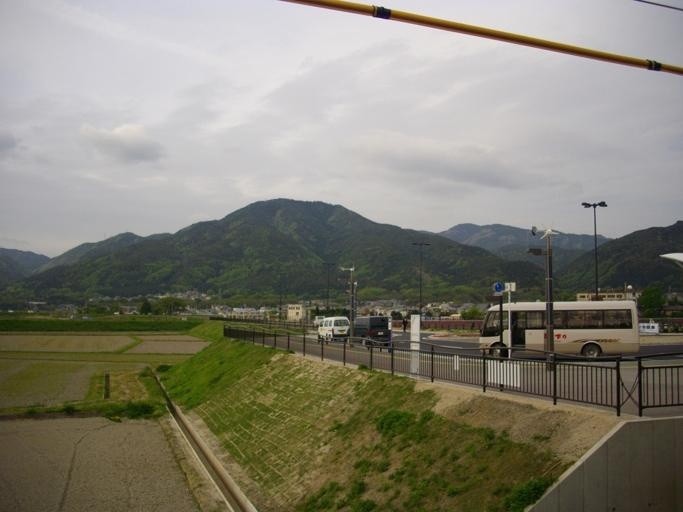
[472,300,640,361]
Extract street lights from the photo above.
[322,262,336,312]
[526,225,559,371]
[339,267,357,347]
[581,201,608,300]
[411,241,432,315]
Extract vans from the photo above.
[313,315,389,347]
[639,323,659,334]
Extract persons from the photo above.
[400,317,408,332]
